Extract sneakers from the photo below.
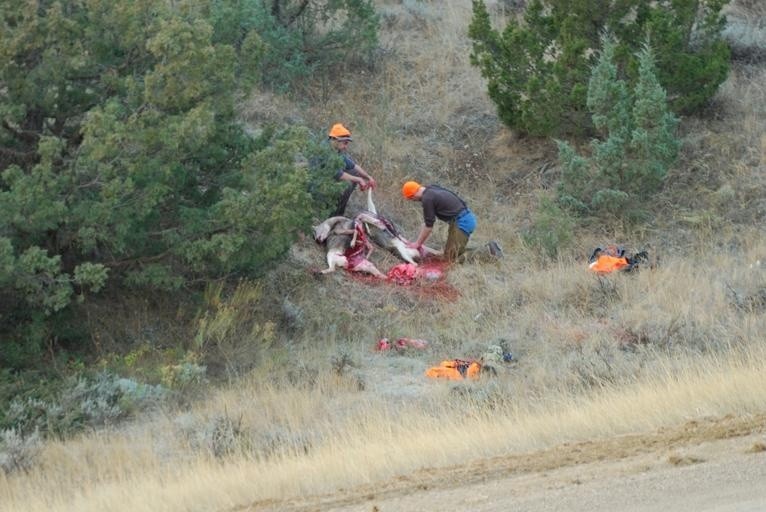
[485,240,504,258]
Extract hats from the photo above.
[401,181,420,200]
[328,122,353,143]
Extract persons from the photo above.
[400,181,504,267]
[306,123,378,218]
